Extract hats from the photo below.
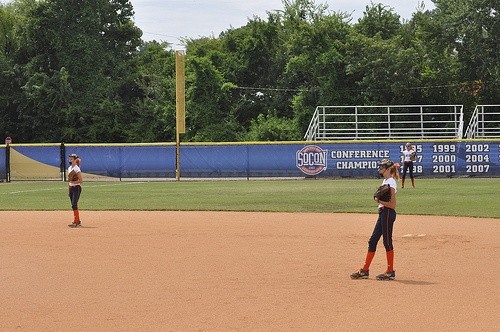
[377,159,393,168]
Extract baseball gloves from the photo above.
[68,170,78,181]
[373,184,391,201]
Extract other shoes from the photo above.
[72,221,81,225]
[411,185,415,188]
[68,223,80,227]
[401,185,404,189]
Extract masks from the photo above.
[69,162,72,165]
[379,172,384,178]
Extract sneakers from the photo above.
[350,269,370,280]
[376,271,396,281]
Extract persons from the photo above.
[67,153,83,227]
[401,142,416,188]
[350,158,397,281]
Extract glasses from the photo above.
[406,145,411,147]
